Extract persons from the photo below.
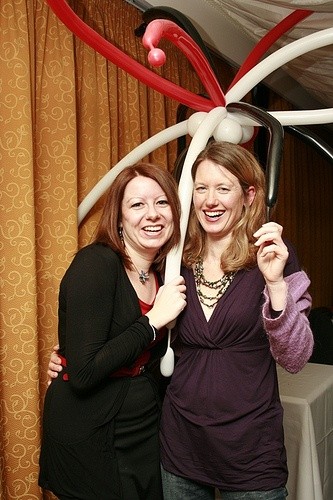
[36,163,189,500]
[48,140,314,500]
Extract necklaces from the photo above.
[129,257,151,284]
[192,249,236,308]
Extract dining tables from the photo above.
[277,363,333,500]
